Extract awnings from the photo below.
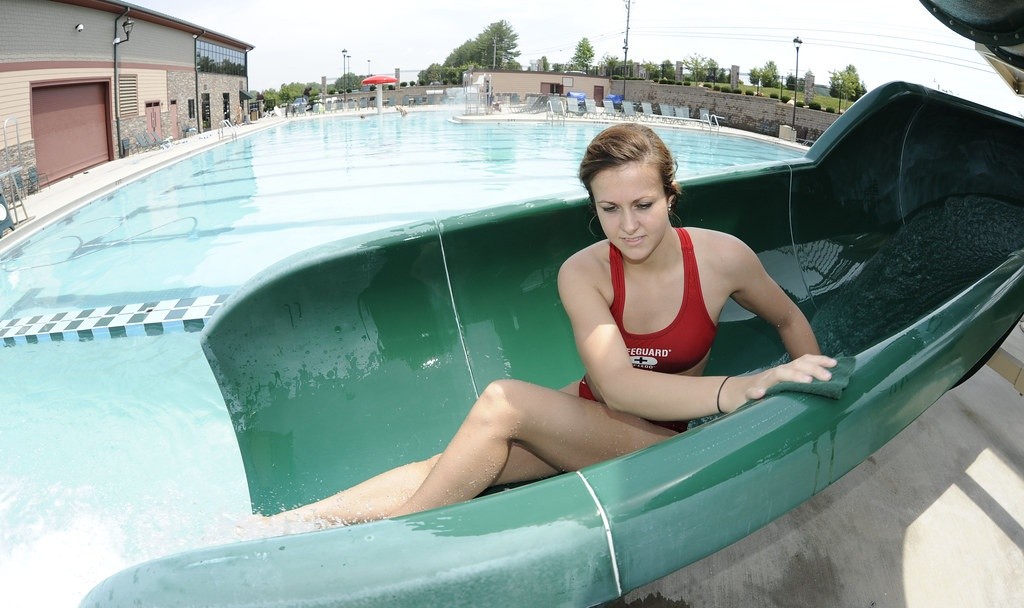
[240,90,257,100]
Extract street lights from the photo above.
[792,36,804,128]
[341,48,348,101]
[347,55,351,74]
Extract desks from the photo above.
[0,166,28,225]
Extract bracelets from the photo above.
[716,376,732,414]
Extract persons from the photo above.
[303,88,314,110]
[485,81,494,114]
[237,122,837,528]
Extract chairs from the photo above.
[495,92,710,128]
[14,166,50,202]
[180,120,198,138]
[120,130,174,153]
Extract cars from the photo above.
[292,97,308,110]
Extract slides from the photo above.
[77,81,1024,608]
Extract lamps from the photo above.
[116,16,134,44]
[192,33,197,38]
